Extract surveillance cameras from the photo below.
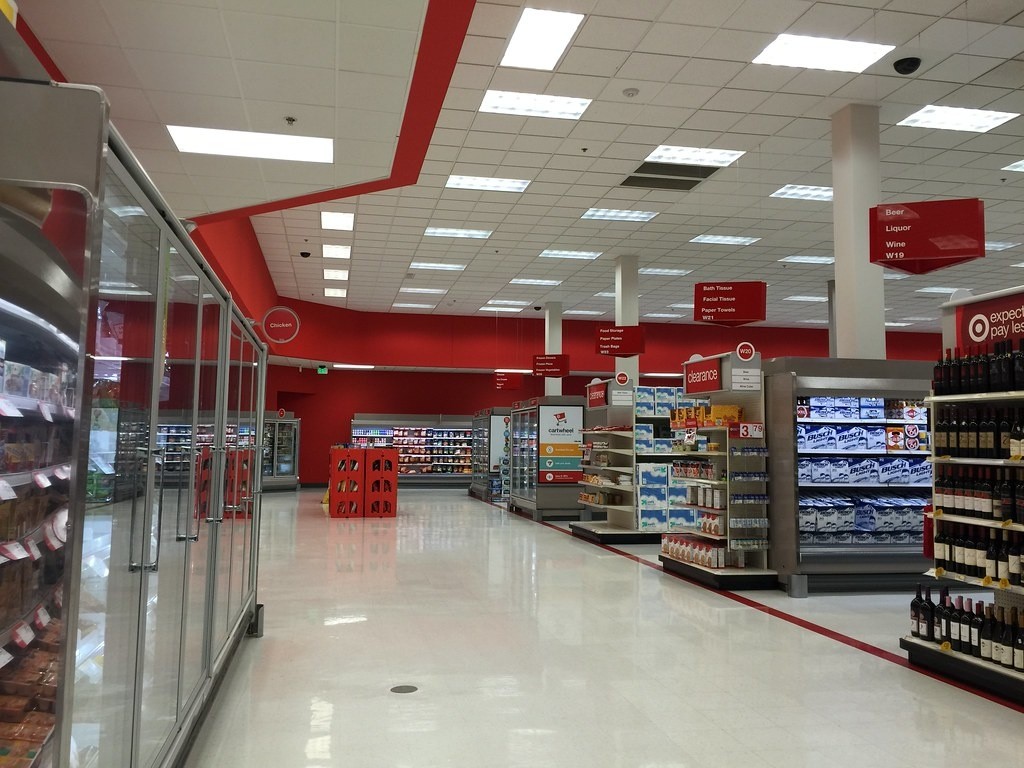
[300,252,312,258]
[534,307,541,310]
[894,57,922,74]
[319,365,326,369]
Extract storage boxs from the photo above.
[488,387,933,570]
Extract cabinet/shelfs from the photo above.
[660,342,992,599]
[365,449,399,518]
[328,448,364,518]
[0,298,78,768]
[468,406,512,501]
[507,395,586,521]
[900,285,1024,708]
[350,419,473,488]
[569,372,683,545]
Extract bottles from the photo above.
[474,426,488,438]
[910,583,1024,673]
[513,440,538,455]
[934,521,1024,586]
[352,437,368,444]
[513,429,537,439]
[473,440,488,454]
[352,427,393,435]
[935,404,1024,460]
[935,464,1024,525]
[933,338,1024,396]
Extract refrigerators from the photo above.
[262,418,302,487]
[509,396,584,510]
[469,407,511,499]
